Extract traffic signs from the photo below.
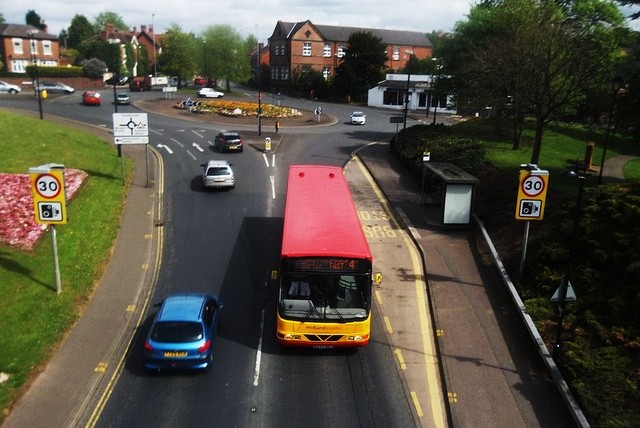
[28,168,66,226]
[515,164,549,220]
[112,112,151,144]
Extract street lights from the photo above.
[598,87,627,178]
[402,49,416,129]
[106,38,123,157]
[24,29,44,120]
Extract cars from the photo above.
[194,77,206,85]
[116,92,131,105]
[350,111,367,125]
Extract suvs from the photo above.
[167,76,189,88]
[0,80,22,95]
[34,80,76,95]
[200,158,237,189]
[82,90,102,107]
[129,79,151,92]
[106,75,126,85]
[141,291,224,376]
[214,131,243,153]
[195,87,225,98]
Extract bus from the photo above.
[270,164,374,351]
